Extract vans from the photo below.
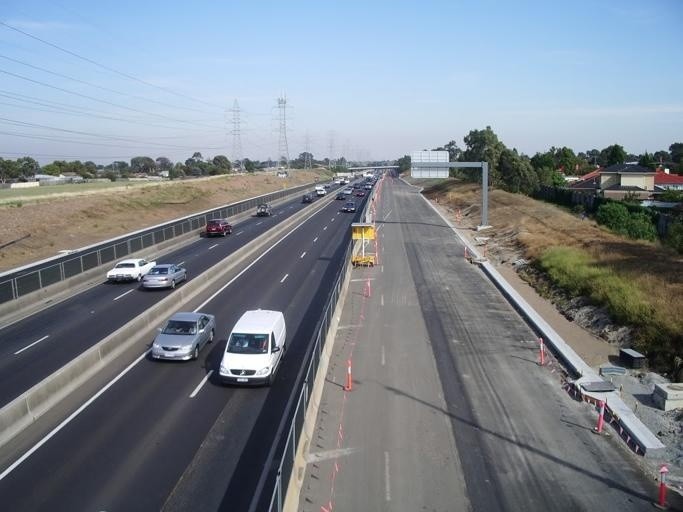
[206,218,232,237]
[217,309,288,387]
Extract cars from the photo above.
[151,312,215,362]
[342,201,356,213]
[107,259,156,281]
[336,176,378,199]
[302,176,349,203]
[141,264,187,290]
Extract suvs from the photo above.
[256,203,272,216]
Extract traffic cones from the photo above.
[343,360,354,391]
[652,466,669,510]
[591,400,607,434]
[535,338,547,366]
